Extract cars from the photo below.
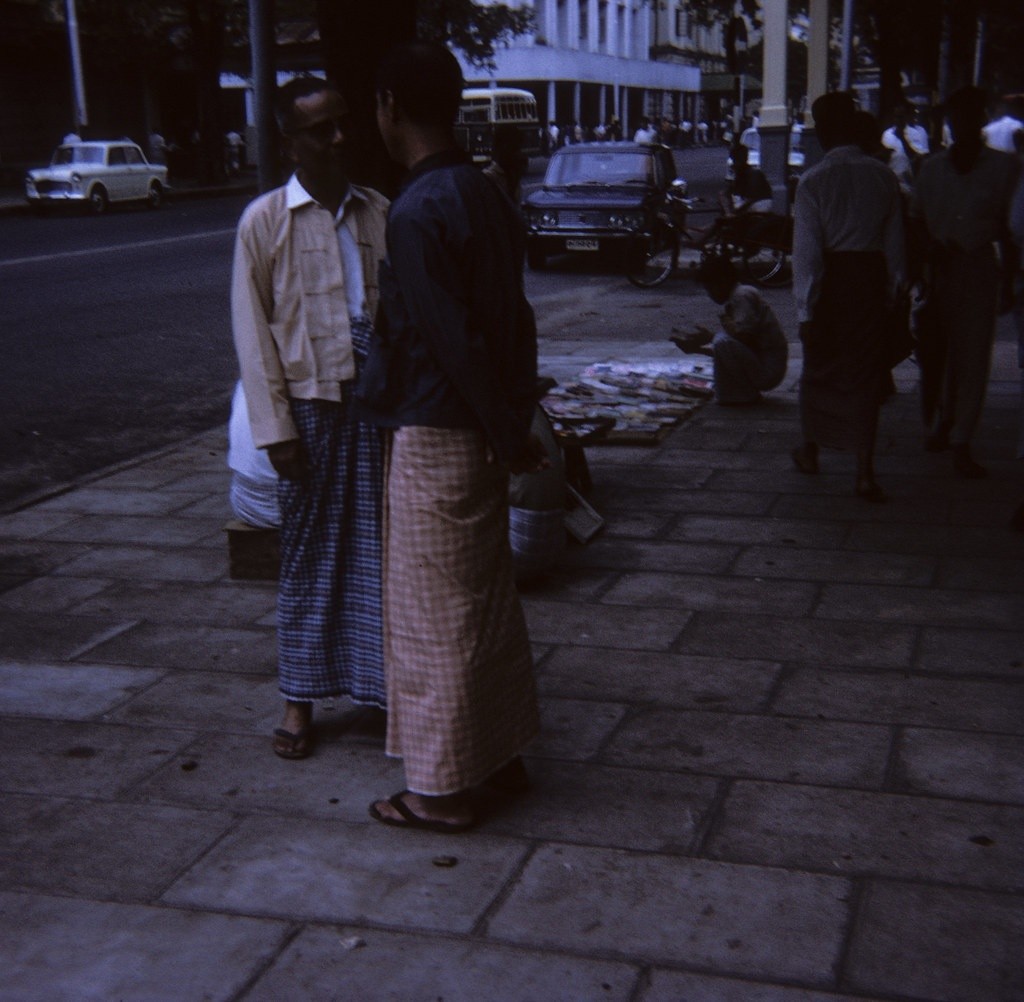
[26,140,168,214]
[520,140,688,271]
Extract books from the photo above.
[668,326,701,345]
[538,359,717,439]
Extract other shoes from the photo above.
[797,460,823,477]
[715,399,757,407]
[860,489,890,505]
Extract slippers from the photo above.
[272,723,315,760]
[369,788,479,835]
[487,754,530,796]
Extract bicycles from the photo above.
[627,177,785,292]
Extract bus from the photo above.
[460,85,542,159]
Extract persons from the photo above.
[229,78,393,759]
[366,42,543,834]
[670,254,789,407]
[221,127,244,171]
[191,127,202,143]
[783,59,1024,370]
[541,112,756,152]
[910,89,1019,474]
[149,130,176,164]
[229,381,593,581]
[720,144,773,213]
[790,92,909,503]
[63,130,82,144]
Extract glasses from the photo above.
[294,113,351,144]
